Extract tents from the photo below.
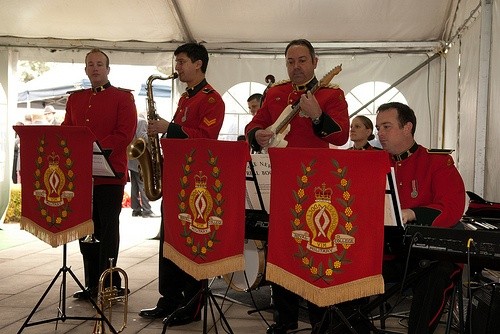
[20,62,170,105]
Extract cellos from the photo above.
[259,75,275,108]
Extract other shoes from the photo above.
[132,211,161,218]
[266,322,298,334]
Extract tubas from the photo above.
[92,257,128,334]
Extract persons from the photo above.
[328,99,467,334]
[241,38,350,334]
[348,114,385,152]
[51,48,138,304]
[125,112,159,218]
[139,42,225,324]
[247,91,263,117]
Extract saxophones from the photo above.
[126,72,181,201]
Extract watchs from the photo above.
[313,113,325,125]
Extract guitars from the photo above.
[253,64,343,154]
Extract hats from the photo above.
[465,191,500,217]
[42,104,56,115]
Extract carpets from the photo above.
[209,276,310,323]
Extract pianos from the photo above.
[244,206,500,334]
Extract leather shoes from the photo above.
[162,303,204,326]
[73,287,98,298]
[116,288,130,296]
[138,296,185,319]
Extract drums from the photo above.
[223,239,270,292]
